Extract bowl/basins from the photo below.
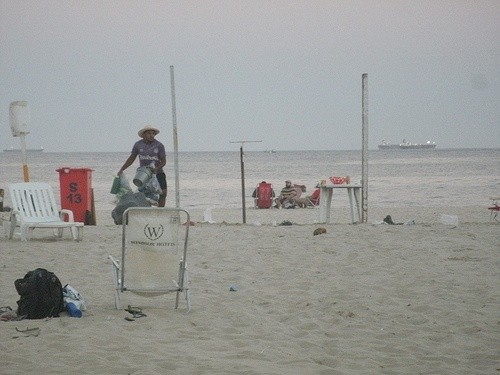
[329,176,345,184]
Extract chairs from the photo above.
[7,182,84,242]
[107,206,191,315]
[488,197,500,223]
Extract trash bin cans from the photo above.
[56,167,94,225]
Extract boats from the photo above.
[377,138,439,151]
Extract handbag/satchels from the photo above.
[62,284,85,311]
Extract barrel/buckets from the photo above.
[133,166,153,186]
[439,213,459,228]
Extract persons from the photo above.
[116,127,167,207]
[275,180,297,208]
[252,181,275,197]
[0,189,9,210]
[306,179,326,204]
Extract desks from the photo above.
[316,184,364,224]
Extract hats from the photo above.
[138,125,160,138]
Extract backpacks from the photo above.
[14,267,63,320]
